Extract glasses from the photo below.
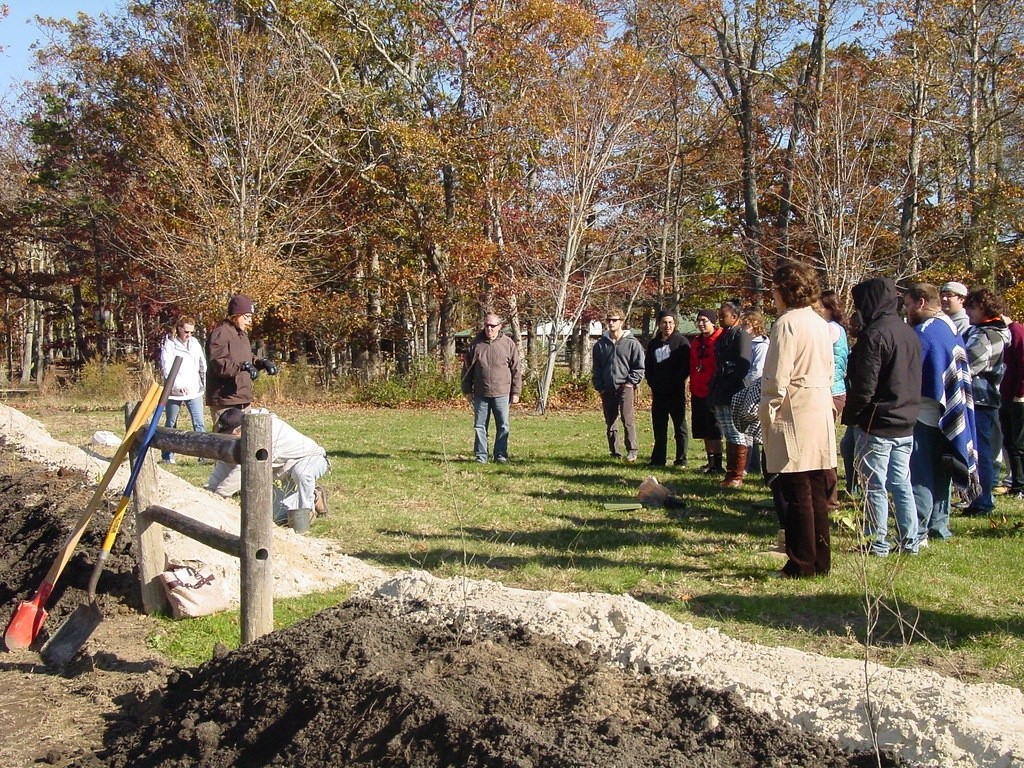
[484,324,500,328]
[180,326,196,335]
[607,319,623,323]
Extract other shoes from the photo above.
[963,505,991,517]
[314,486,327,515]
[954,501,969,508]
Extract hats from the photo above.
[699,309,717,325]
[229,295,254,314]
[942,282,967,298]
[217,408,245,435]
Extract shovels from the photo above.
[40,356,184,665]
[5,382,164,652]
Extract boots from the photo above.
[719,441,747,486]
[702,452,724,474]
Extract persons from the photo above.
[161,314,207,464]
[205,294,277,433]
[739,310,771,475]
[644,309,691,469]
[204,408,331,527]
[710,299,753,488]
[758,264,838,580]
[689,309,725,473]
[592,308,645,461]
[812,278,1024,557]
[460,314,522,465]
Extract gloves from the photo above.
[240,362,258,381]
[261,360,278,377]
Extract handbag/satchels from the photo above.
[161,559,228,620]
